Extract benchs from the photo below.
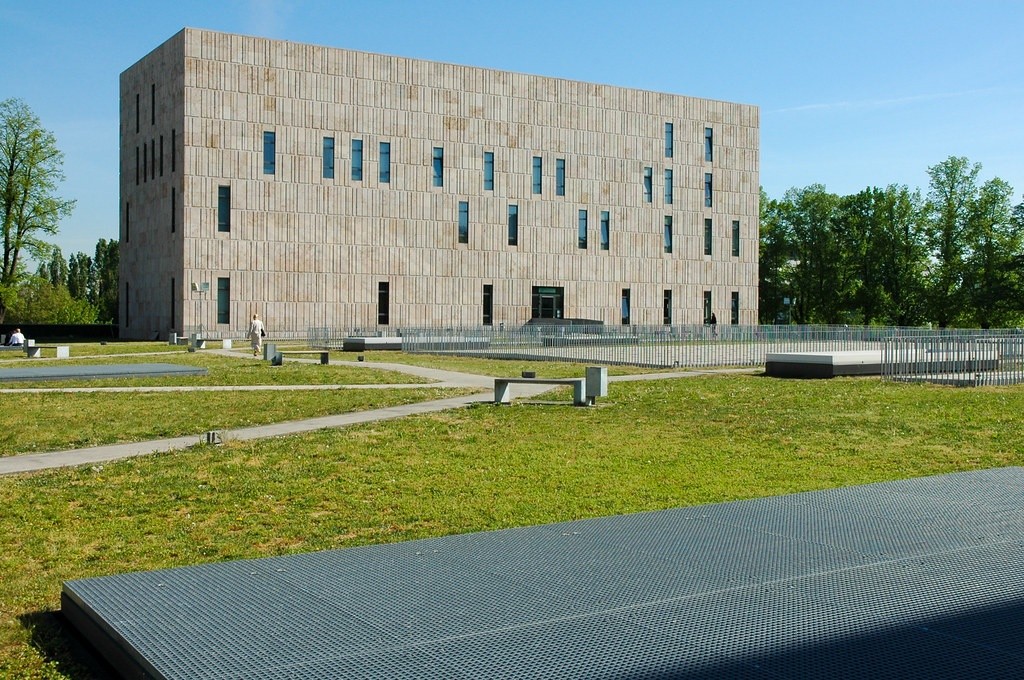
[275,351,329,367]
[494,378,587,404]
[28,346,70,359]
[197,338,231,349]
[177,337,189,345]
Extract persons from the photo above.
[710,313,717,336]
[248,314,264,356]
[4,329,26,346]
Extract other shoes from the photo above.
[254,351,257,356]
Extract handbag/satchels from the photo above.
[261,328,265,336]
